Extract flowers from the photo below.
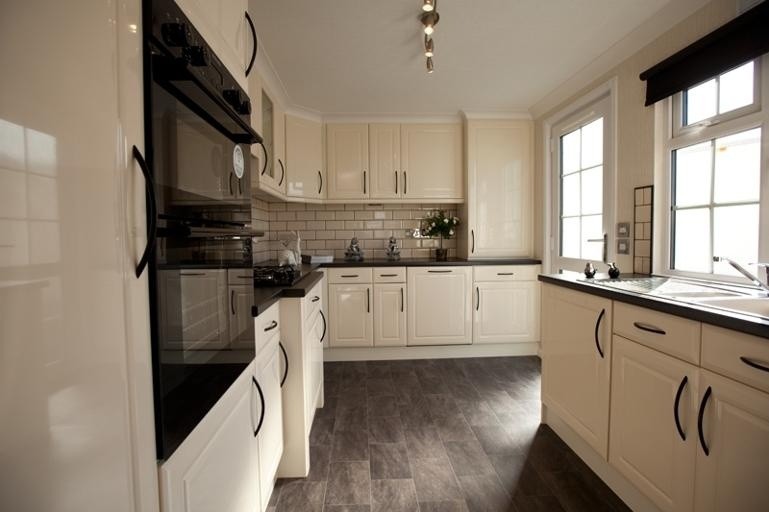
[418,206,460,247]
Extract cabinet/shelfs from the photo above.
[609,301,769,512]
[458,117,536,262]
[248,64,286,203]
[328,122,464,204]
[156,362,259,511]
[281,279,326,479]
[283,113,324,205]
[173,0,258,96]
[254,301,285,512]
[326,267,407,362]
[407,267,473,359]
[474,269,541,360]
[539,281,612,487]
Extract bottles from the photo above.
[609,263,619,279]
[584,263,595,278]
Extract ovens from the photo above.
[143,1,264,465]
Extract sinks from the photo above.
[697,298,769,325]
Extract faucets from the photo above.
[713,255,769,297]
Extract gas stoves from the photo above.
[253,264,301,289]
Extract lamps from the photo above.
[420,0,441,74]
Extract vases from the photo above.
[436,248,448,263]
